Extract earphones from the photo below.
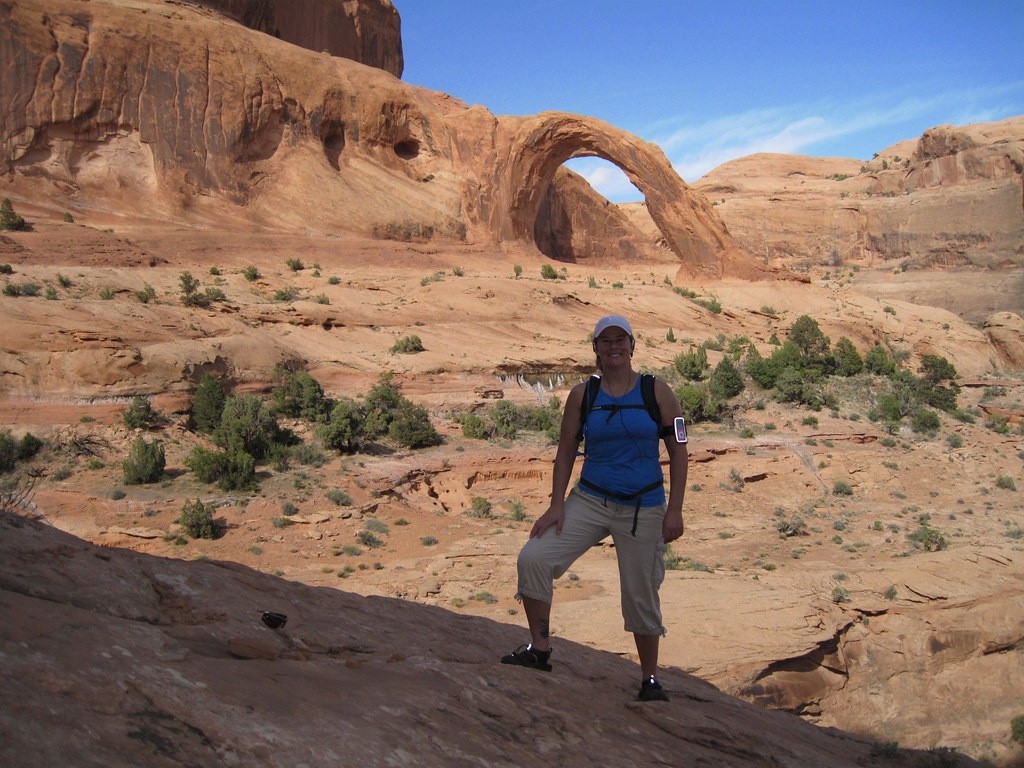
[631,340,634,350]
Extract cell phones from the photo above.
[674,416,687,443]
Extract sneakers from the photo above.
[637,674,670,702]
[500,642,553,672]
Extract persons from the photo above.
[502,317,689,700]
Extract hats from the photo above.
[593,316,633,339]
